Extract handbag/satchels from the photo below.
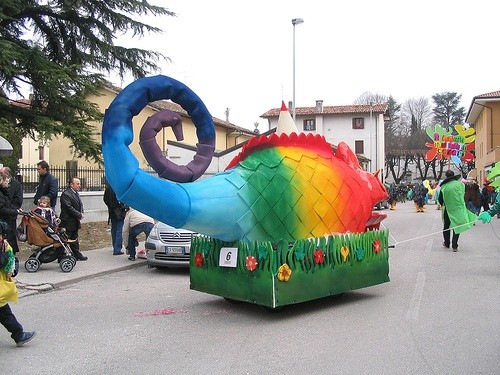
[115,204,130,220]
[0,268,19,308]
[16,220,27,242]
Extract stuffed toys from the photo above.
[477,161,500,223]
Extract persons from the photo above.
[0,221,37,347]
[0,167,23,255]
[104,185,155,259]
[33,196,60,232]
[33,160,58,210]
[60,177,88,261]
[383,181,406,208]
[428,179,441,204]
[436,169,477,252]
[407,182,429,212]
[462,175,497,214]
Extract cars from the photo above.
[374,199,390,210]
[144,221,210,269]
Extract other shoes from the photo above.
[71,253,87,261]
[120,251,124,255]
[128,256,135,261]
[453,248,458,252]
[15,330,37,346]
[442,243,450,249]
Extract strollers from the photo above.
[16,208,77,273]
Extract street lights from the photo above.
[292,17,305,125]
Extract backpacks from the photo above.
[0,237,19,277]
